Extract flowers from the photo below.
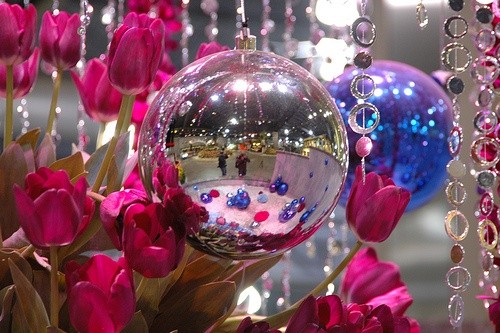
[0,1,423,332]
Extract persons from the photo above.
[218,151,229,177]
[236,154,251,177]
